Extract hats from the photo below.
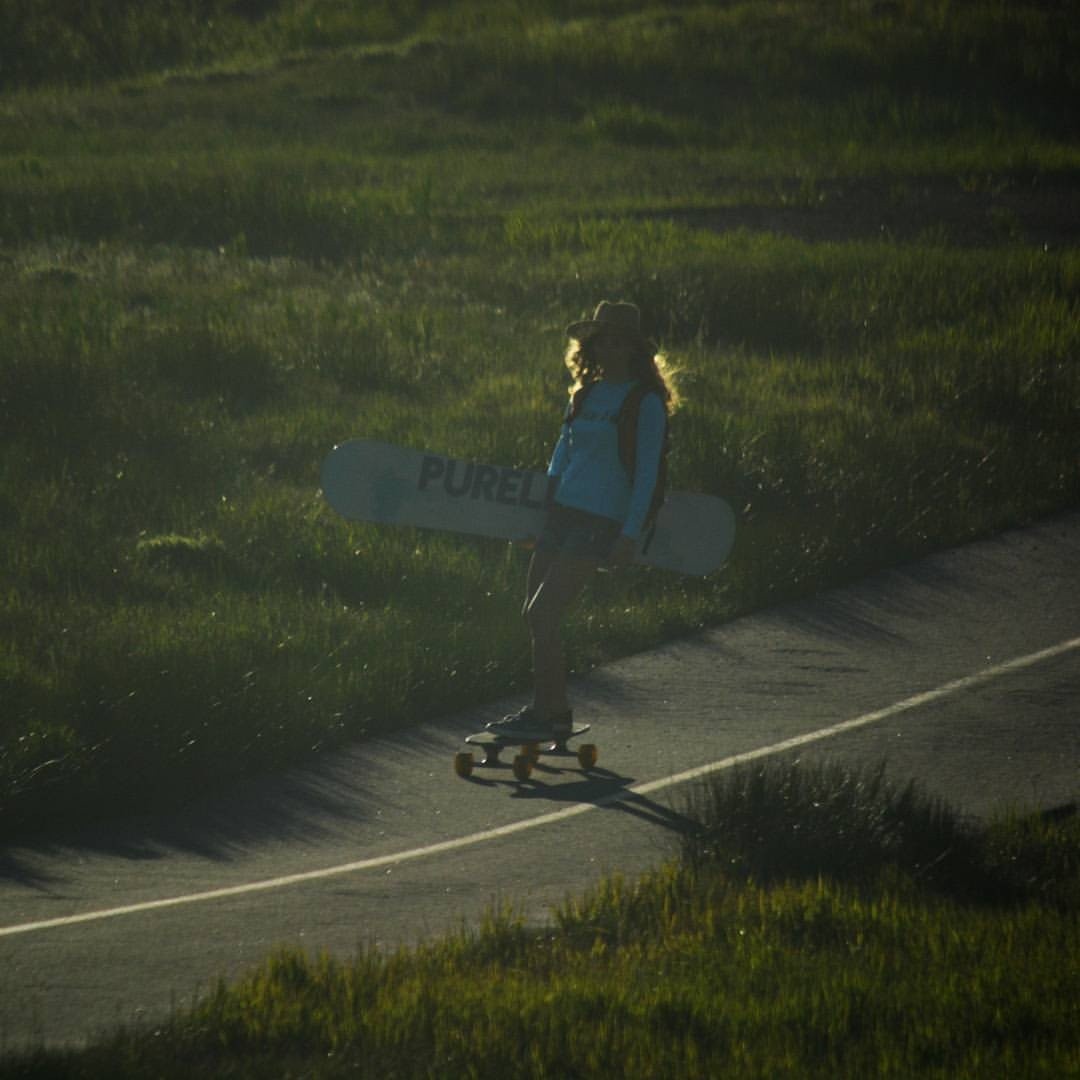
[566,300,658,356]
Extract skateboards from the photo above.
[454,721,598,783]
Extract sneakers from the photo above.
[485,705,552,739]
[545,704,572,733]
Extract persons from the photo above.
[485,300,668,739]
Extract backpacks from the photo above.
[567,380,670,524]
